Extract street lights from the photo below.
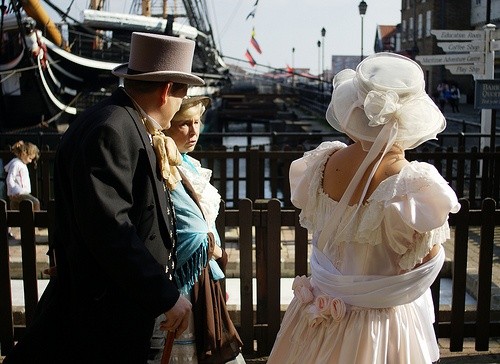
[317,27,326,76]
[358,0,368,62]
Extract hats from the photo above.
[180,95,212,106]
[331,52,448,145]
[111,32,205,87]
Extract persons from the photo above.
[3,32,204,364]
[3,139,41,211]
[266,52,462,364]
[149,93,249,364]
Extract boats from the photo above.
[0,0,351,209]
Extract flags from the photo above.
[250,37,262,54]
[245,49,257,67]
